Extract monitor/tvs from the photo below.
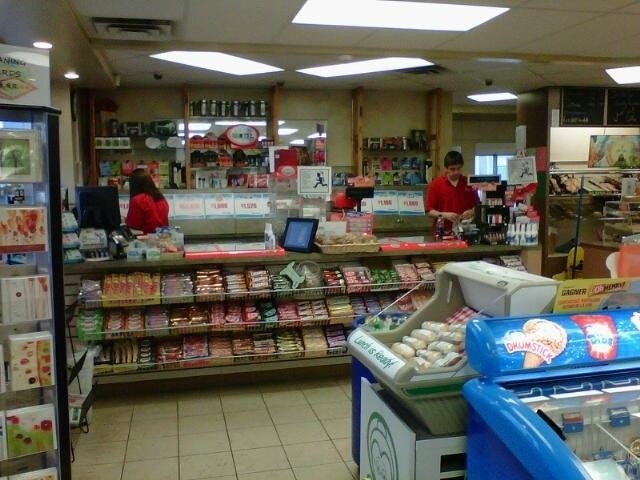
[74,185,122,232]
[281,217,320,252]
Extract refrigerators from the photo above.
[460,307,640,479]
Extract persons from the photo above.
[124,168,169,234]
[425,151,480,238]
[232,149,248,168]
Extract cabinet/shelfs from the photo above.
[182,86,278,188]
[517,89,640,279]
[60,235,526,386]
[1,102,71,480]
[359,138,433,186]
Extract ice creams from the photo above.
[523,318,568,369]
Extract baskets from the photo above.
[315,243,381,255]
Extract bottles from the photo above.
[437,214,444,242]
[402,137,407,150]
[190,100,267,116]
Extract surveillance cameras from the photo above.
[154,72,163,80]
[277,79,286,85]
[486,79,493,86]
